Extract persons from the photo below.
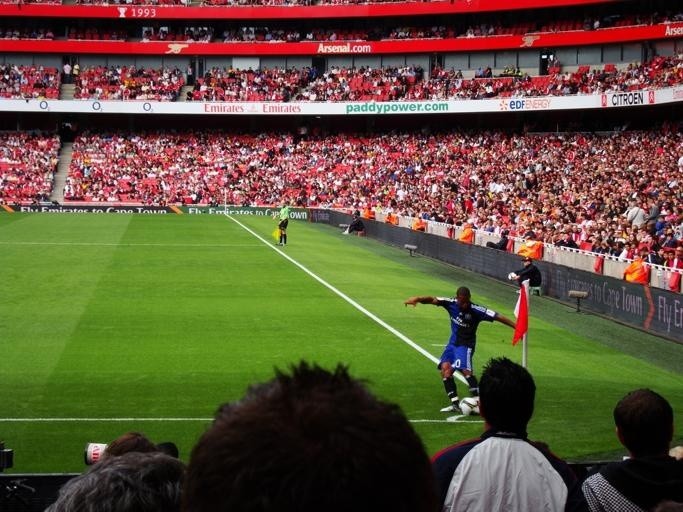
[525,2,683,277]
[404,285,524,414]
[427,357,578,512]
[514,259,541,289]
[579,388,683,512]
[186,364,445,512]
[0,0,525,249]
[39,428,192,512]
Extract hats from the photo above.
[520,256,532,261]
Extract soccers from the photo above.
[508,272,517,281]
[459,396,480,415]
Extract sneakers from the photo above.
[440,404,461,413]
[275,242,283,246]
